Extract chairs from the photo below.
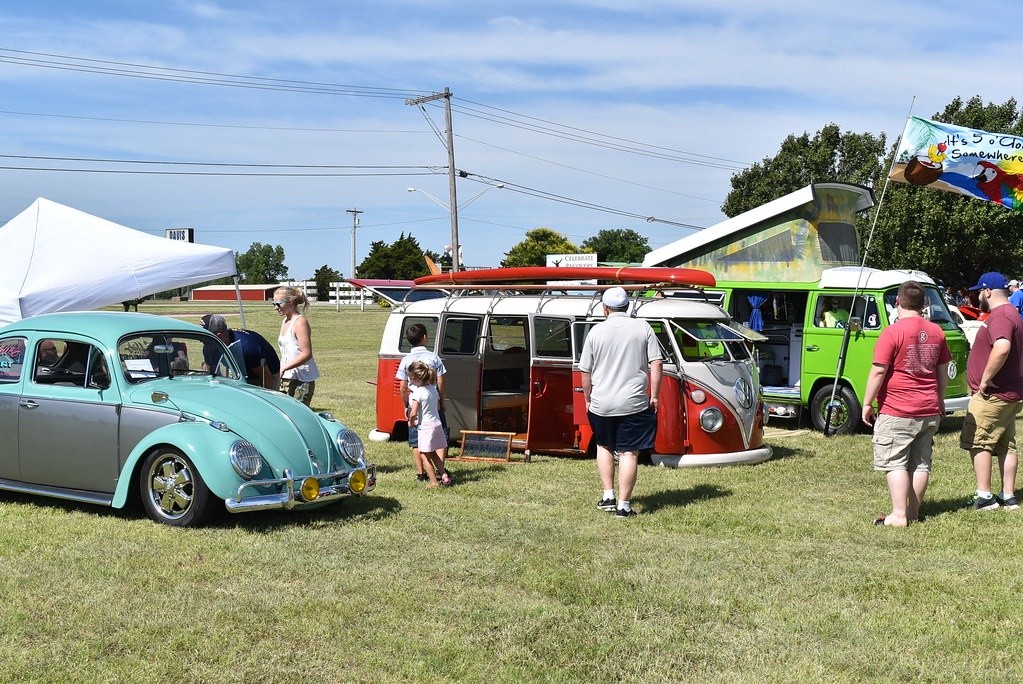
[815,296,829,327]
[150,343,190,379]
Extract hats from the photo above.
[969,271,1009,290]
[199,314,227,333]
[602,287,628,307]
[1008,279,1020,287]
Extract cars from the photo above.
[945,304,987,351]
[0,311,377,529]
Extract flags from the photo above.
[891,116,1023,212]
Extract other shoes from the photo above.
[434,469,449,479]
[441,477,451,485]
[416,473,429,480]
[426,483,439,488]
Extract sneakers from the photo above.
[597,498,617,512]
[994,493,1019,509]
[961,492,999,511]
[615,508,637,517]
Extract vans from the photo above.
[345,268,774,470]
[632,181,973,438]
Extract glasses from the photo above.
[272,301,289,308]
[977,287,993,295]
[43,346,56,352]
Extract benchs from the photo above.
[37,370,103,391]
[481,355,530,429]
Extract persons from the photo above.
[396,323,454,482]
[199,314,280,390]
[946,286,975,306]
[862,280,952,527]
[272,286,321,406]
[578,287,663,518]
[144,338,186,375]
[960,272,1023,511]
[40,340,59,365]
[1008,279,1023,319]
[408,361,451,487]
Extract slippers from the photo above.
[873,517,885,526]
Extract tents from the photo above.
[0,197,247,328]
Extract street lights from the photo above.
[406,182,504,296]
